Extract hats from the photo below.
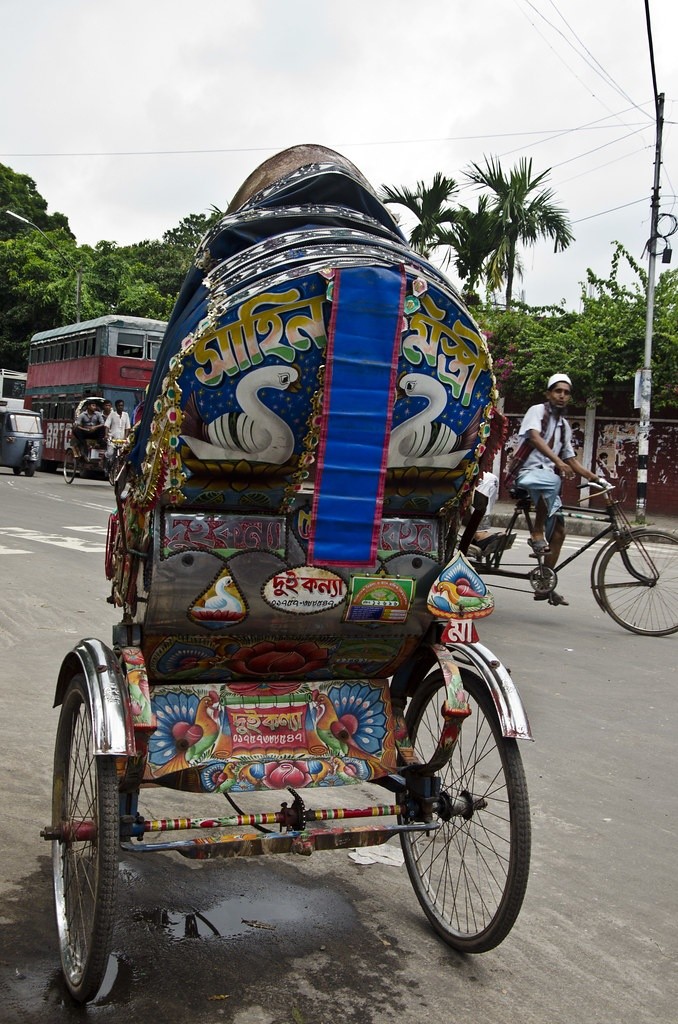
[547,373,572,390]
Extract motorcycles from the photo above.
[0,409,45,477]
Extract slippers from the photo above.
[534,590,570,606]
[527,538,552,556]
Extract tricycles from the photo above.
[40,138,533,1003]
[62,397,130,487]
[453,477,678,637]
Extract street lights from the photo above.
[7,210,81,321]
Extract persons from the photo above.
[472,470,500,530]
[101,400,114,420]
[513,373,599,604]
[104,400,130,469]
[73,401,104,455]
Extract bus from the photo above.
[23,314,169,477]
[0,369,27,412]
